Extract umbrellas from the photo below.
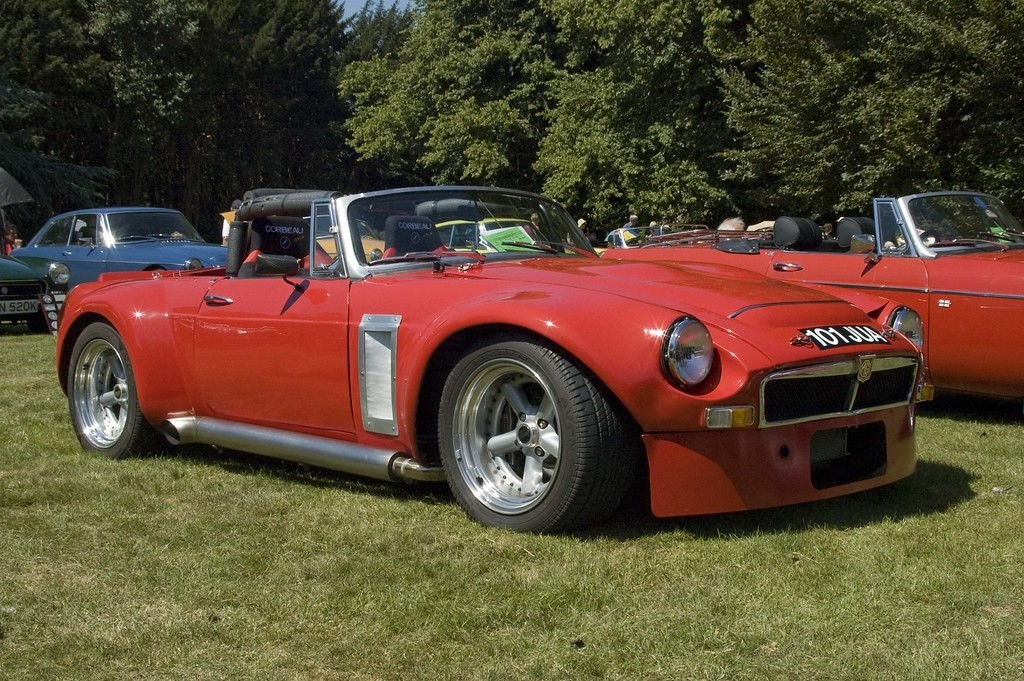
[604,228,639,241]
[0,168,35,210]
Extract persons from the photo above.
[576,218,588,238]
[624,215,672,240]
[523,213,540,240]
[222,199,242,246]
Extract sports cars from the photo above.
[56,181,931,537]
[638,190,1023,413]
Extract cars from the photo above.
[427,218,610,259]
[220,209,387,266]
[0,257,71,336]
[8,205,229,324]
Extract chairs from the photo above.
[838,217,875,253]
[242,216,333,270]
[381,216,456,262]
[773,217,823,251]
